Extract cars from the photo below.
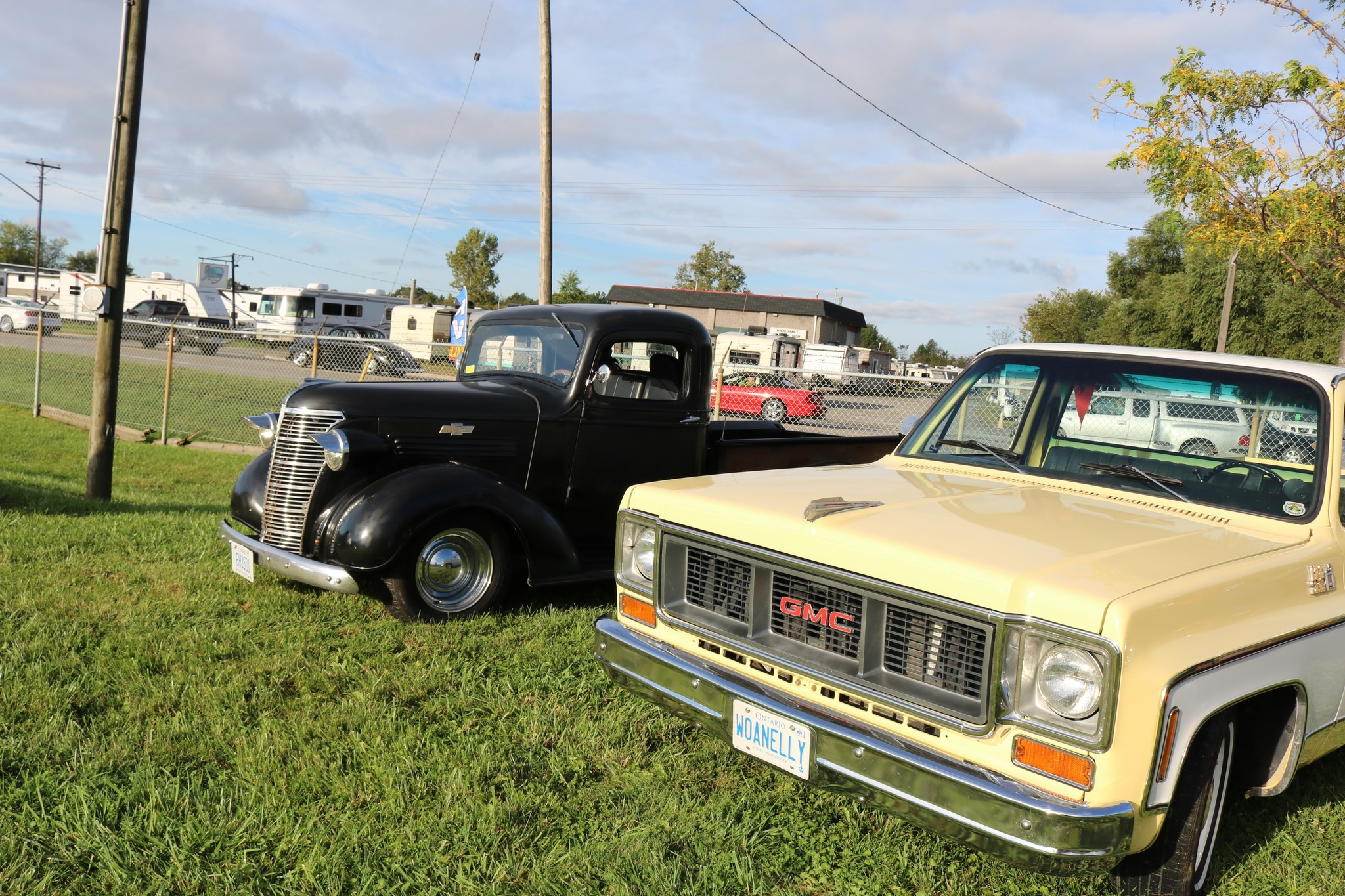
[285,325,425,378]
[0,297,62,336]
[710,372,828,423]
[1243,409,1318,464]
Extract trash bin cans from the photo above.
[1004,405,1015,418]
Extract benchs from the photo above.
[593,350,685,402]
[1039,446,1313,498]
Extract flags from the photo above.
[451,287,465,345]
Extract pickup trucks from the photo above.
[219,305,919,625]
[1056,392,1251,460]
[120,300,231,356]
[594,341,1345,896]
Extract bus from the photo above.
[256,283,411,347]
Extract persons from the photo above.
[740,376,756,386]
